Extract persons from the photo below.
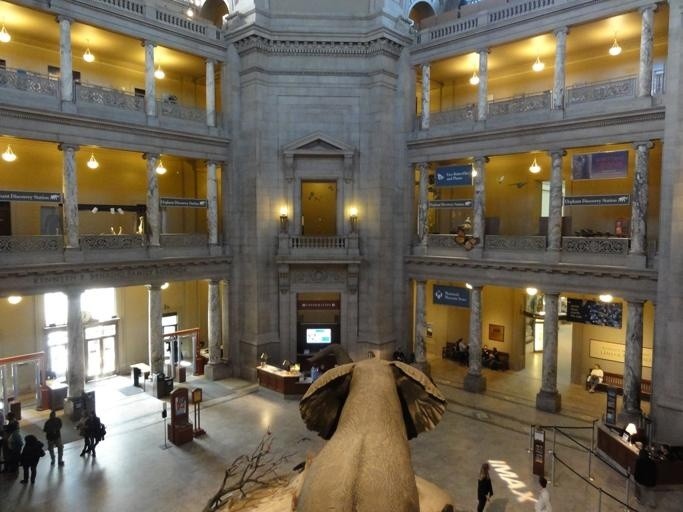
[476,462,493,512]
[455,337,467,367]
[488,347,500,368]
[0,391,105,485]
[482,344,489,366]
[586,363,604,393]
[533,476,553,512]
[391,345,407,364]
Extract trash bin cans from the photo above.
[153,371,174,399]
[40,206,63,234]
[64,391,96,422]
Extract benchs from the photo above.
[586,368,652,402]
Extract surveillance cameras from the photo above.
[91,206,99,215]
[116,207,125,215]
[110,207,115,215]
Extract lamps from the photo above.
[0,23,11,44]
[470,65,479,85]
[86,146,99,169]
[154,65,166,80]
[529,150,542,174]
[155,154,168,175]
[1,137,17,162]
[533,56,543,71]
[82,48,95,63]
[609,31,622,55]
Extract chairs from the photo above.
[442,342,510,371]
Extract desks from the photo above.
[45,378,69,412]
[256,364,300,395]
[130,363,150,392]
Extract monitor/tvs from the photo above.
[306,328,332,345]
